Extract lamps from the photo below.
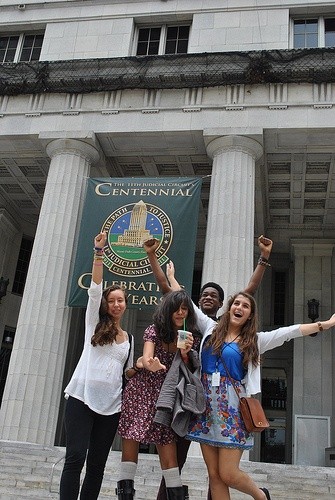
[0,276,10,303]
[307,298,320,338]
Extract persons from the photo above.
[59,230,145,500]
[114,289,203,500]
[143,234,274,500]
[166,260,334,500]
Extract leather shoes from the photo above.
[258,488,270,500]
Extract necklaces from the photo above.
[173,337,178,346]
[228,330,241,341]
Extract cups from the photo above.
[176,329,193,349]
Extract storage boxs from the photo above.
[325,447,335,467]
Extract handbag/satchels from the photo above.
[239,397,269,433]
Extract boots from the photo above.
[166,485,189,500]
[114,479,135,500]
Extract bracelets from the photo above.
[92,245,105,266]
[316,321,323,331]
[258,257,271,266]
